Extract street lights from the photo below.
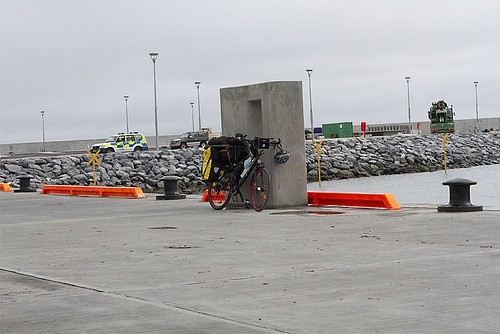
[148,52,159,151]
[405,77,411,123]
[306,69,314,140]
[190,102,194,132]
[194,81,202,130]
[41,110,44,151]
[473,81,479,119]
[123,94,129,133]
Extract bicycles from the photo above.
[201,137,281,212]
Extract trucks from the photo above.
[427,100,456,133]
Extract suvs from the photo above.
[92,132,149,153]
[170,131,210,149]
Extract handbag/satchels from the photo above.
[201,136,250,182]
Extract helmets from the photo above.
[274,150,290,164]
